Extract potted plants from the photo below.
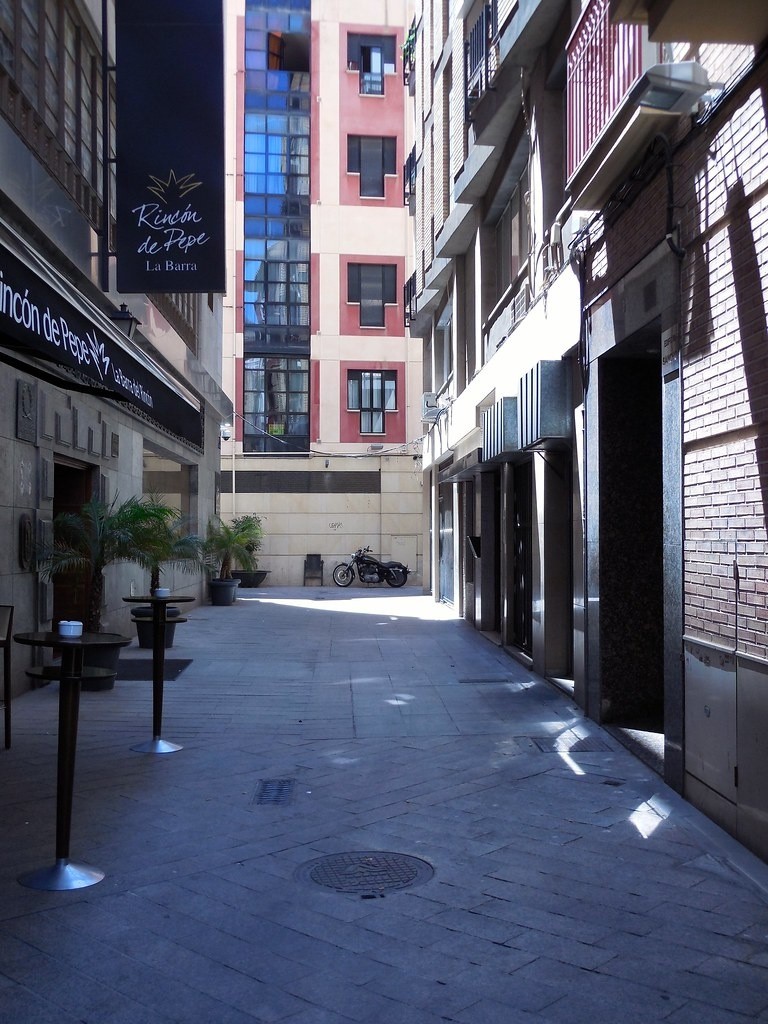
[102,525,197,648]
[204,513,268,604]
[26,489,182,691]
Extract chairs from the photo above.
[303,553,323,586]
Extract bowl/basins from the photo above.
[231,570,272,588]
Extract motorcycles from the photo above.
[333,545,411,588]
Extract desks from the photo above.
[121,595,197,754]
[11,632,134,891]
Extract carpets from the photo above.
[113,658,196,681]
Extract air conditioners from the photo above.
[420,392,436,420]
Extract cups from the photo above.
[58,621,83,639]
[155,589,169,598]
